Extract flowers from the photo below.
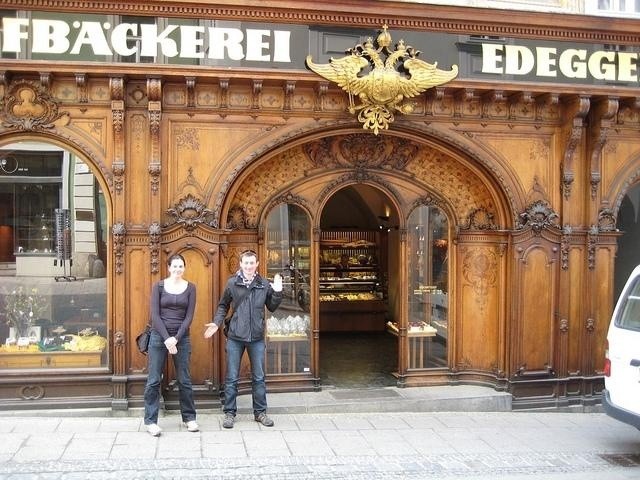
[0,283,47,336]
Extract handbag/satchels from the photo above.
[223,317,231,338]
[136,330,151,354]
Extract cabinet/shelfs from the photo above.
[289,240,383,305]
[11,182,63,257]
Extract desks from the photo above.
[0,339,104,368]
[265,330,308,374]
[385,319,438,379]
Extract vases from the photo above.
[16,336,30,350]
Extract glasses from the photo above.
[242,250,256,255]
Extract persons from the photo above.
[203,250,283,427]
[144,254,200,436]
[334,255,349,277]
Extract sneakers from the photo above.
[222,413,234,428]
[184,421,200,432]
[255,415,274,426]
[146,423,163,436]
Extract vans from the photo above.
[598,258,640,431]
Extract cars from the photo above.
[272,267,310,300]
